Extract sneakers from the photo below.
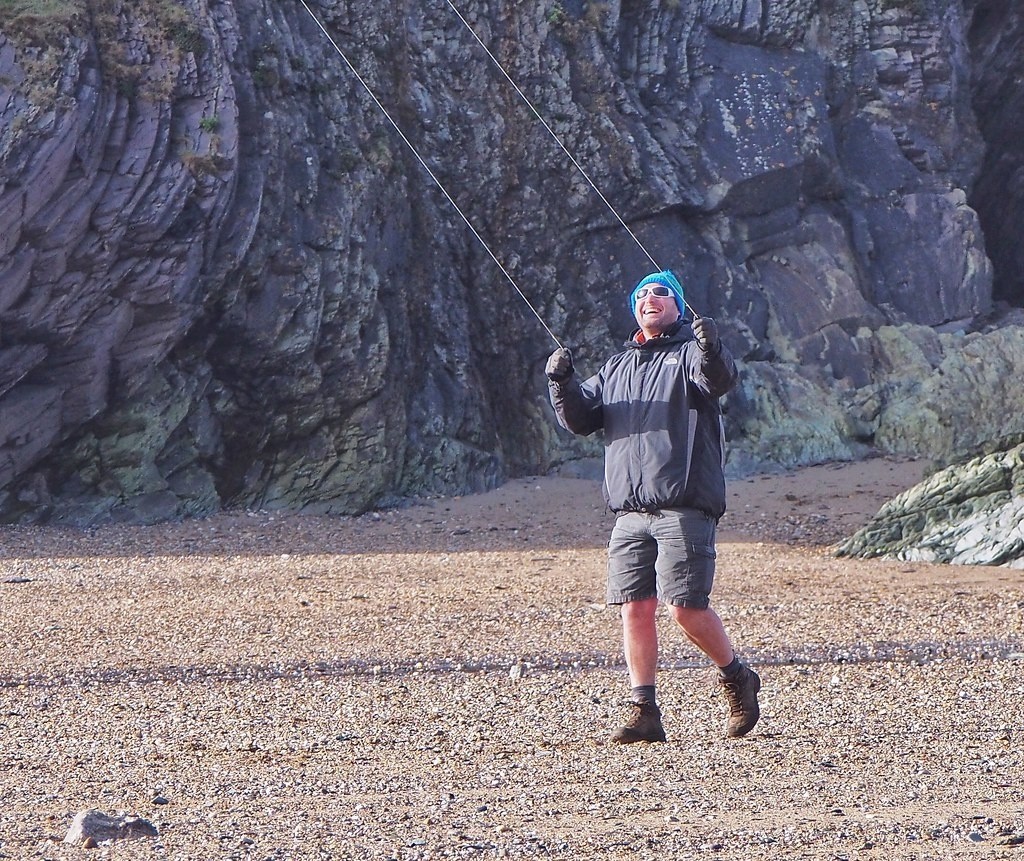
[613,699,665,744]
[714,663,760,736]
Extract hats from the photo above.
[631,271,685,321]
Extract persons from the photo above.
[544,268,763,745]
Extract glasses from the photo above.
[634,286,675,301]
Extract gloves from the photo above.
[544,347,575,382]
[691,314,719,355]
